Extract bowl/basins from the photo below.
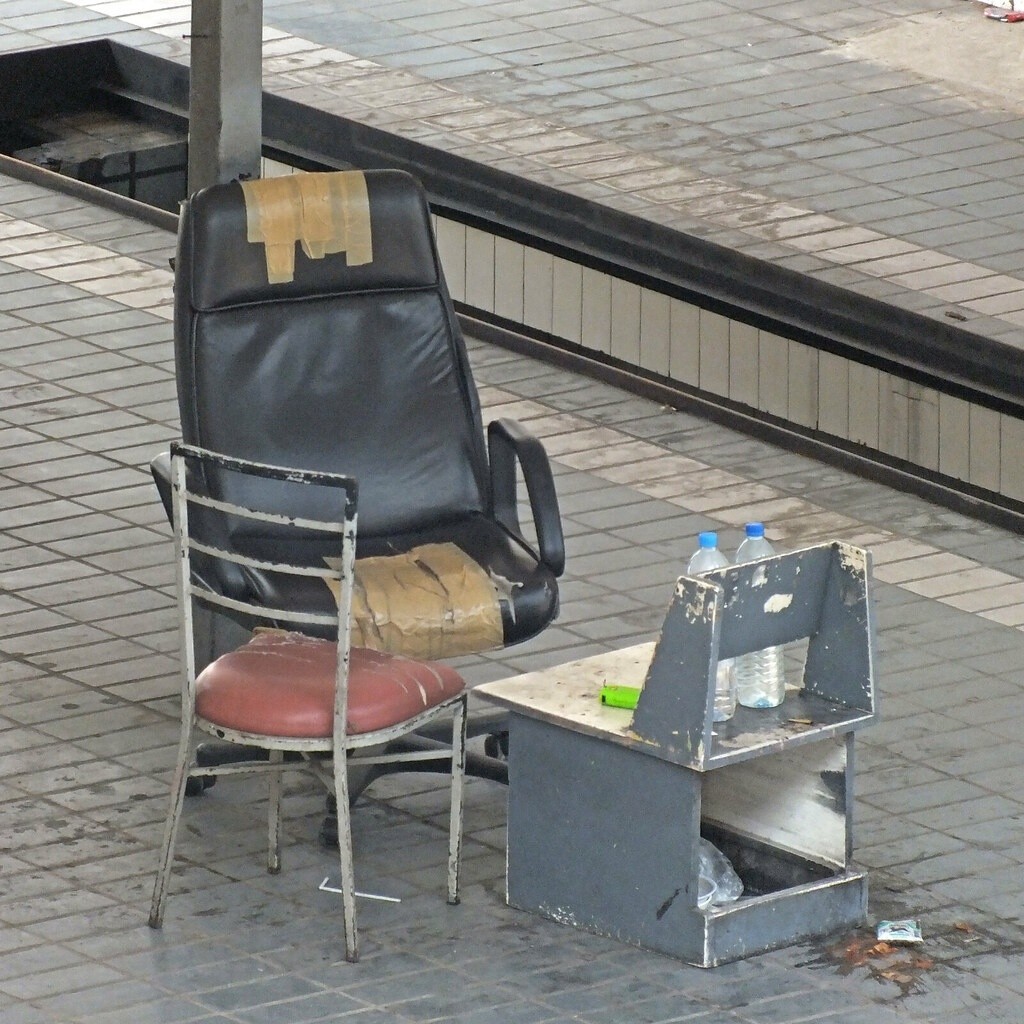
[697,874,717,909]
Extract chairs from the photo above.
[470,542,877,970]
[149,170,565,844]
[147,442,467,963]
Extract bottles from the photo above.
[732,522,786,706]
[687,532,737,723]
